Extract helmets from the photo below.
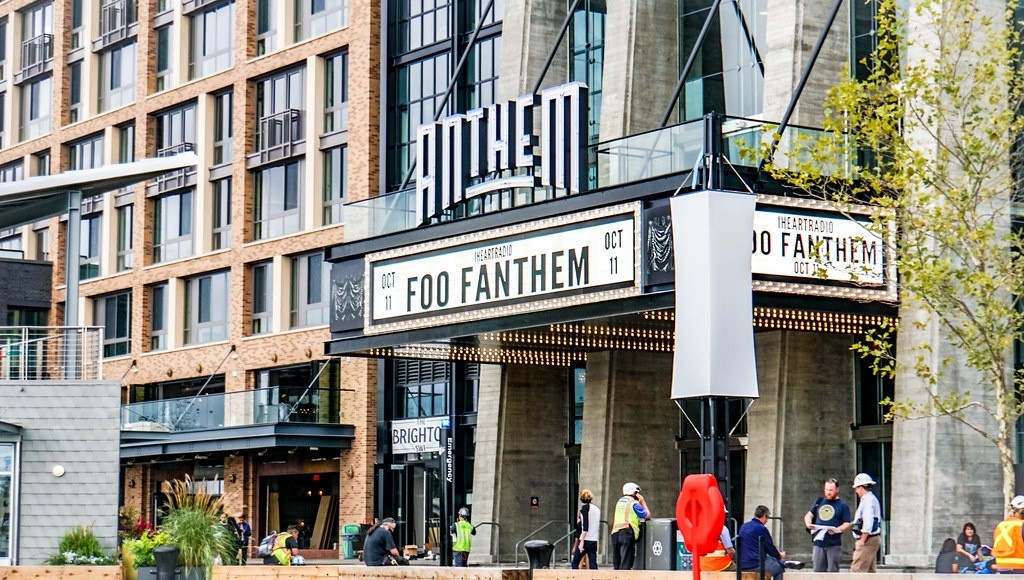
[852,473,876,489]
[723,504,729,513]
[457,507,469,516]
[1010,495,1024,509]
[291,556,305,565]
[623,483,641,496]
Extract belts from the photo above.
[857,533,880,540]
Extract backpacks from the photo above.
[258,531,290,558]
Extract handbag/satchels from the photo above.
[806,497,823,534]
[229,517,240,541]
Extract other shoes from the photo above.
[783,560,805,569]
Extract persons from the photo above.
[571,488,601,570]
[364,517,409,566]
[736,505,804,580]
[264,525,300,566]
[955,523,984,570]
[218,511,252,566]
[610,482,651,570]
[934,538,961,573]
[992,495,1024,574]
[805,477,851,571]
[699,505,736,571]
[451,507,476,567]
[850,472,883,574]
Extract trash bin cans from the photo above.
[632,517,693,570]
[359,524,373,550]
[341,524,360,559]
